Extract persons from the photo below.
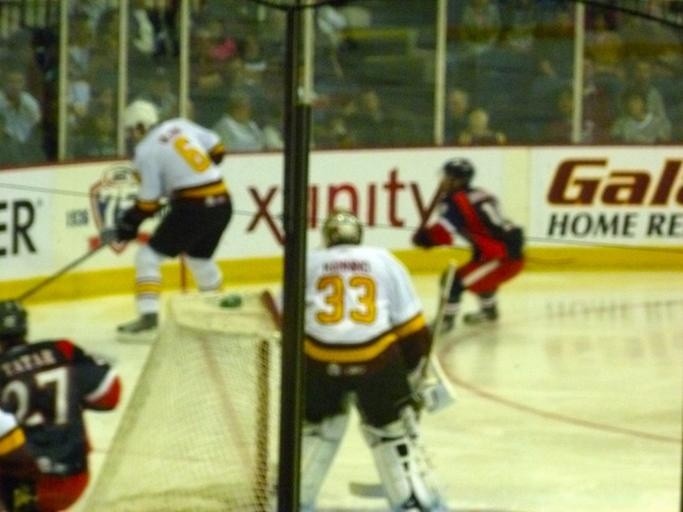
[273,208,445,512]
[409,159,528,336]
[0,1,681,169]
[110,99,234,334]
[0,298,122,512]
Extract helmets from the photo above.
[125,99,158,129]
[0,300,28,337]
[443,158,473,178]
[323,210,361,245]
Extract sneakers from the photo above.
[428,314,454,332]
[464,304,497,323]
[118,316,158,333]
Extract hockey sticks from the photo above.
[348,258,457,499]
[0,223,118,306]
